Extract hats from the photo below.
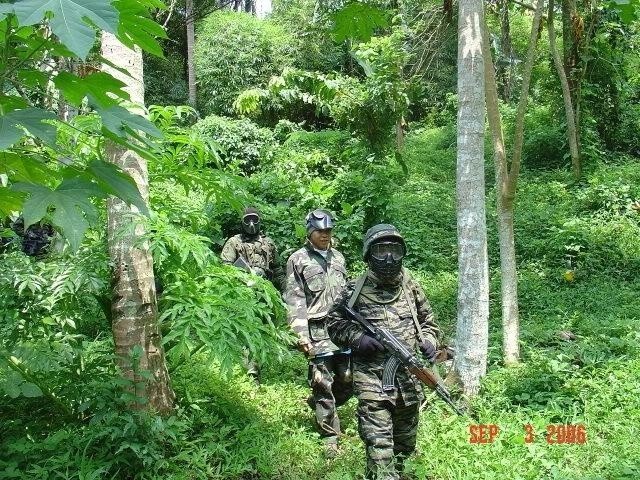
[240,208,259,219]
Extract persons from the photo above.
[323,223,447,479]
[219,207,286,385]
[282,210,355,457]
[1,205,58,262]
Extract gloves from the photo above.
[419,339,438,364]
[358,333,386,355]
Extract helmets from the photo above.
[306,209,333,238]
[362,223,407,261]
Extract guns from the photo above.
[341,304,468,417]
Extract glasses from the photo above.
[370,242,406,260]
[312,209,334,220]
[243,215,259,224]
